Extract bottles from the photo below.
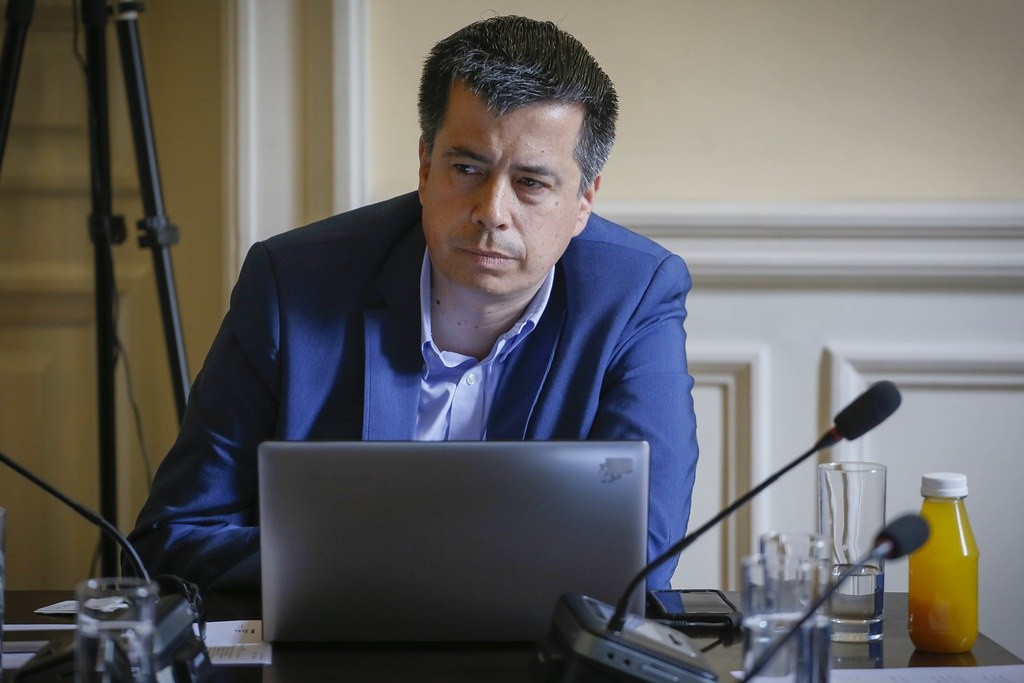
[909,471,979,652]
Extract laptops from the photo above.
[257,442,649,646]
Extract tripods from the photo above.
[0,0,191,591]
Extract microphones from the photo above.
[740,515,932,683]
[551,380,901,683]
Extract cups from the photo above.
[75,577,158,683]
[739,556,815,683]
[761,531,823,613]
[818,462,886,642]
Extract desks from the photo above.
[0,590,1024,683]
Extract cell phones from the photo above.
[649,589,741,632]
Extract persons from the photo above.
[115,13,705,619]
